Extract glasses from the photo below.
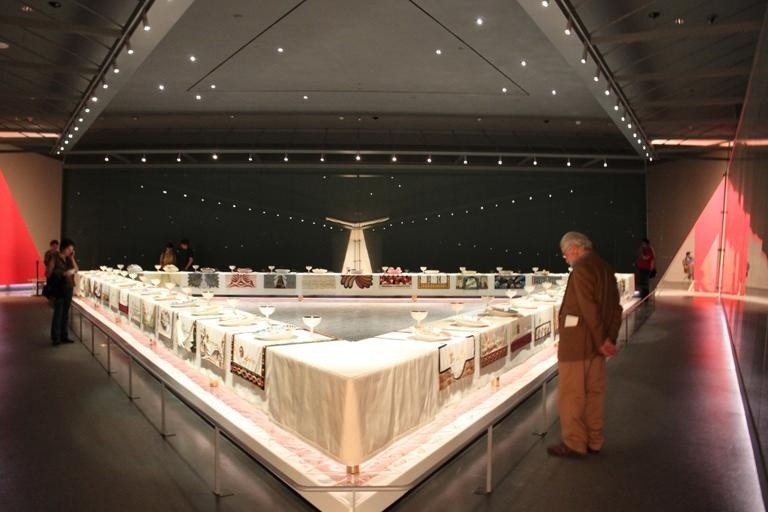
[562,246,571,259]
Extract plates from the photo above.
[312,269,327,273]
[412,334,450,341]
[253,333,298,341]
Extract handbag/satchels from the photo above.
[636,255,655,271]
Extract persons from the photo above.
[176,239,198,270]
[633,239,655,302]
[683,250,695,287]
[46,240,79,345]
[159,244,177,270]
[546,232,624,458]
[43,240,60,270]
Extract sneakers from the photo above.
[547,442,588,459]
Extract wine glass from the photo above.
[100,264,322,337]
[382,267,563,327]
